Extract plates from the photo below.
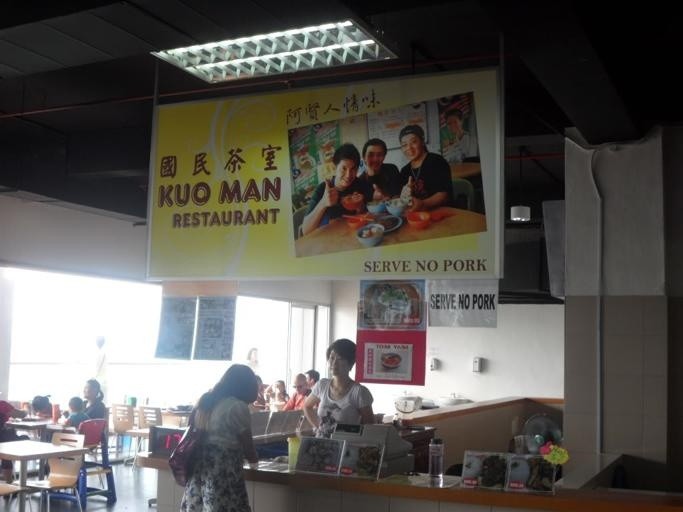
[377,215,402,233]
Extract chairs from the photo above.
[247,398,318,437]
[0,400,163,512]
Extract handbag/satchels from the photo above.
[168,418,200,486]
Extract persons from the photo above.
[248,368,321,415]
[302,338,375,434]
[178,363,259,512]
[301,107,469,238]
[0,378,108,501]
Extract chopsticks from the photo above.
[342,213,375,223]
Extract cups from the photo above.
[127,397,137,407]
[52,404,59,422]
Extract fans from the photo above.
[523,412,562,454]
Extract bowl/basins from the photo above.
[356,224,383,247]
[366,202,385,213]
[386,199,407,216]
[407,210,430,231]
[341,192,366,211]
[347,215,373,231]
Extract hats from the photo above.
[399,125,424,141]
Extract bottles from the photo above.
[428,438,443,477]
[286,436,299,470]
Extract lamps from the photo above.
[148,17,398,88]
[508,147,531,223]
